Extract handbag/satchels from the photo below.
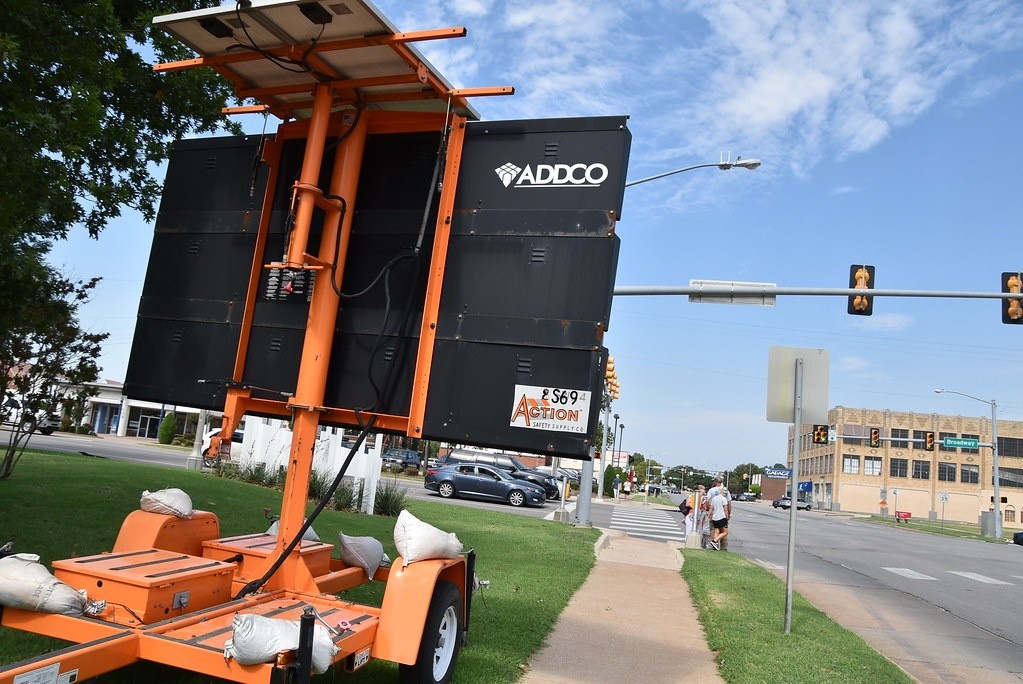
[678,499,692,516]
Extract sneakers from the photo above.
[710,540,719,551]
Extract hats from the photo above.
[711,476,722,483]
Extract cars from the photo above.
[730,494,812,511]
[423,449,598,506]
[639,485,681,494]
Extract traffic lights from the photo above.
[813,424,829,444]
[870,428,880,448]
[606,356,620,399]
[925,431,934,451]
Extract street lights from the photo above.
[934,389,1003,539]
[612,413,620,467]
[617,423,625,468]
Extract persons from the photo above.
[611,473,631,502]
[685,477,732,552]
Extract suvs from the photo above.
[0,388,62,436]
[381,450,419,473]
[200,428,244,468]
